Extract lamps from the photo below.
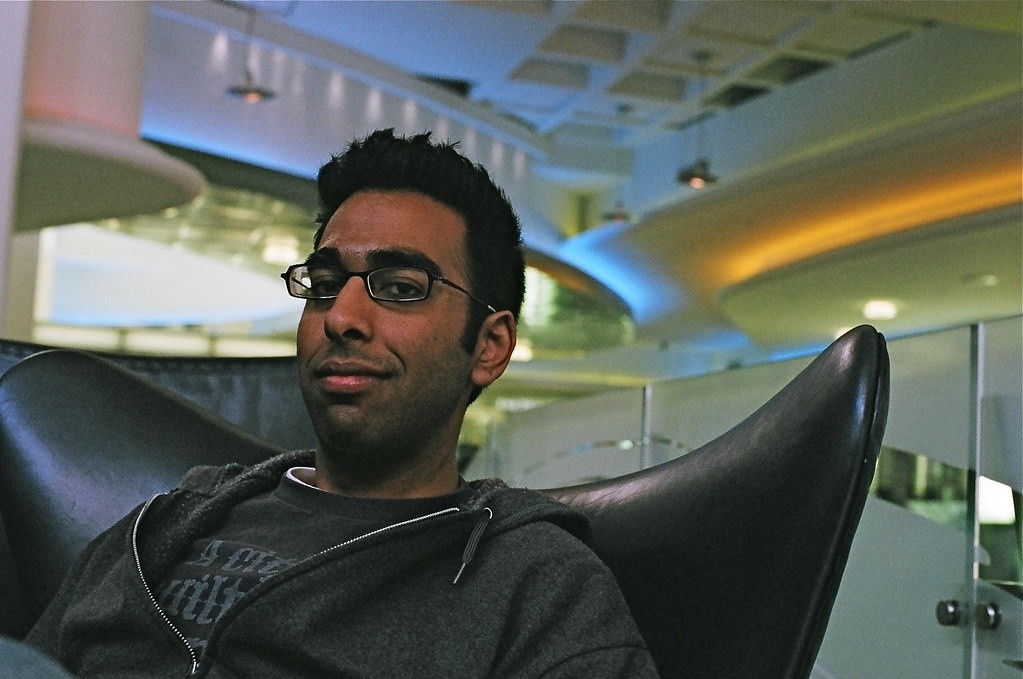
[677,47,718,188]
[602,107,634,220]
[227,7,277,103]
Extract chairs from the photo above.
[1,325,897,678]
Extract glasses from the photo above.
[280,264,496,314]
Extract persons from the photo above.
[0,127,659,679]
